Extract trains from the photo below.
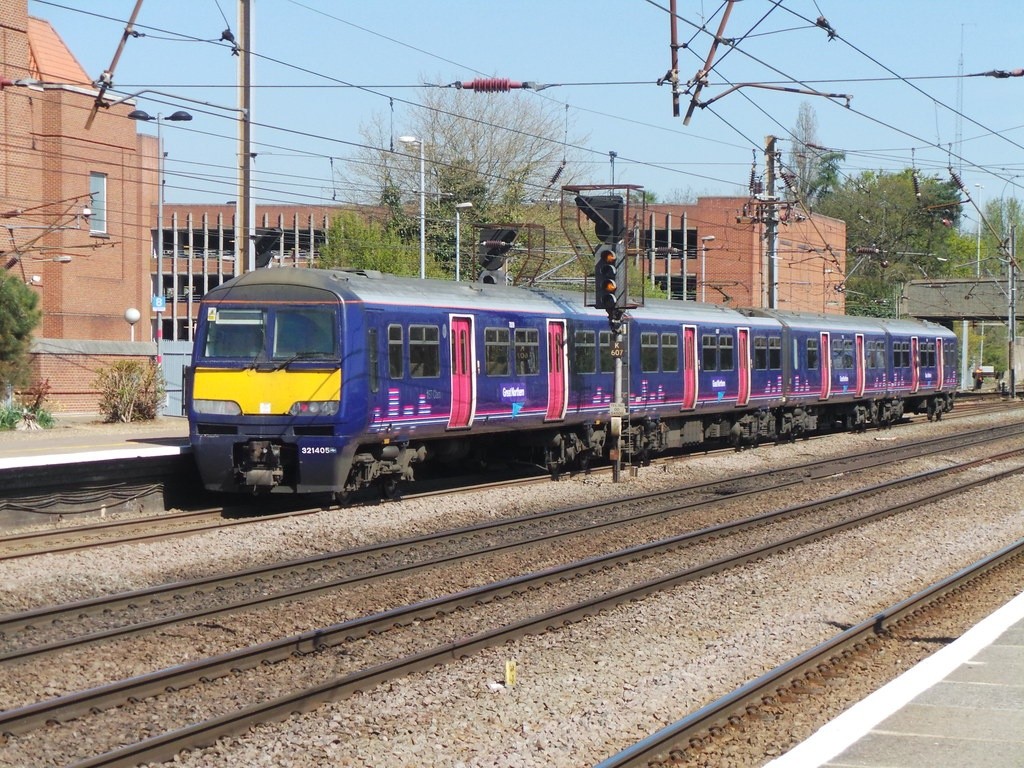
[181,266,961,508]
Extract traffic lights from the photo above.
[595,244,626,309]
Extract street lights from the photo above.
[701,235,716,302]
[127,109,194,423]
[455,202,474,282]
[398,135,426,280]
